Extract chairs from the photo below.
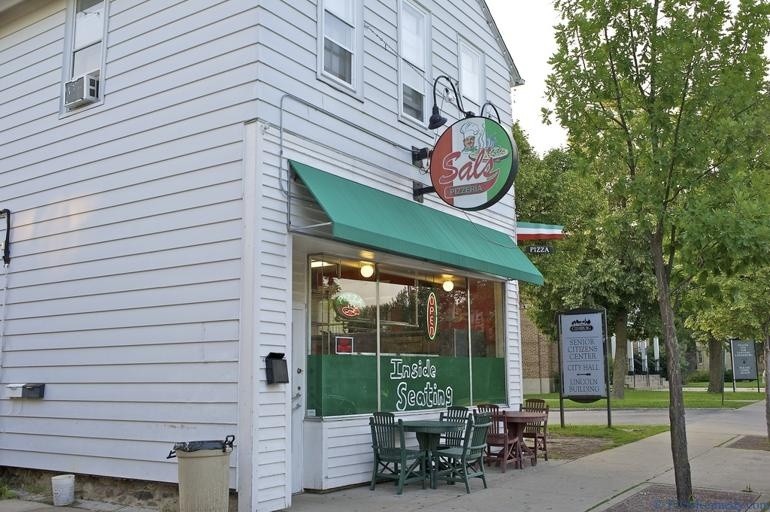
[438,406,472,483]
[432,413,493,493]
[369,411,427,495]
[478,397,552,474]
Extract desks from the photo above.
[391,421,466,488]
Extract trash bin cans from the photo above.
[167,435,236,511]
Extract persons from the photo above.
[459,121,482,155]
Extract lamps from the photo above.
[428,75,474,129]
[360,265,374,278]
[442,281,454,292]
